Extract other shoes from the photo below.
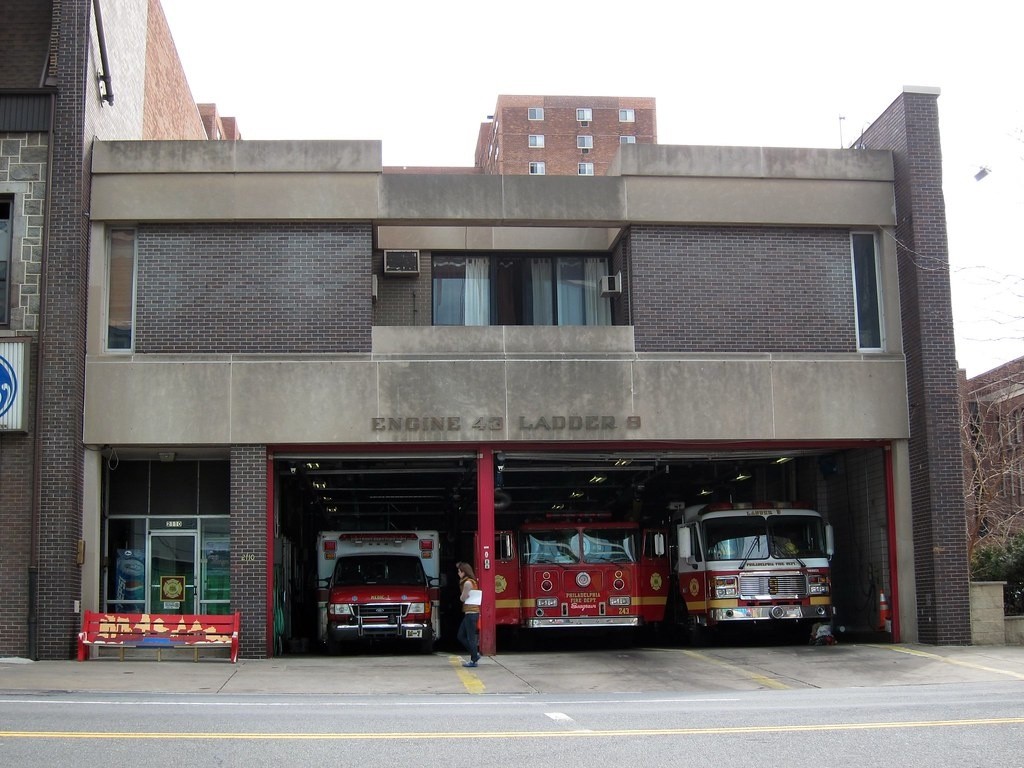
[462,661,479,667]
[476,652,480,660]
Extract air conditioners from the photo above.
[383,249,420,277]
[598,276,619,297]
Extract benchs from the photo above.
[77,611,242,665]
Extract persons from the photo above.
[456,562,482,667]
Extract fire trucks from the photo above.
[475,505,673,641]
[670,496,836,643]
[315,526,443,648]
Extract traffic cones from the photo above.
[878,587,892,628]
